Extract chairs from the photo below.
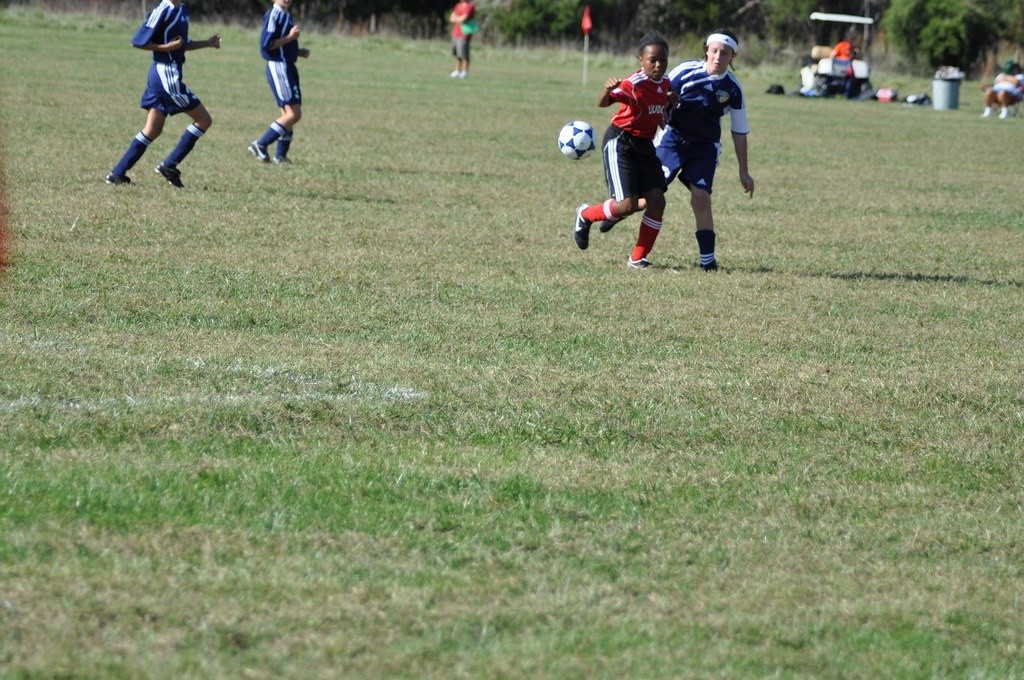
[983,67,1024,116]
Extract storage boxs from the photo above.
[876,88,898,102]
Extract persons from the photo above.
[448,1,475,80]
[828,36,855,59]
[574,27,756,270]
[247,0,311,164]
[979,58,1023,122]
[103,0,222,189]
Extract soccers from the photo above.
[556,120,597,161]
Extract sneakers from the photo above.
[105,171,131,186]
[273,155,292,165]
[248,140,270,162]
[599,217,626,233]
[154,161,185,188]
[574,203,590,250]
[699,260,722,271]
[627,256,652,271]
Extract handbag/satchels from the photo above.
[461,18,480,35]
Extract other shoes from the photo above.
[980,109,994,119]
[458,71,468,78]
[998,108,1009,119]
[451,70,460,78]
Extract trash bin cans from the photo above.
[932,74,962,108]
